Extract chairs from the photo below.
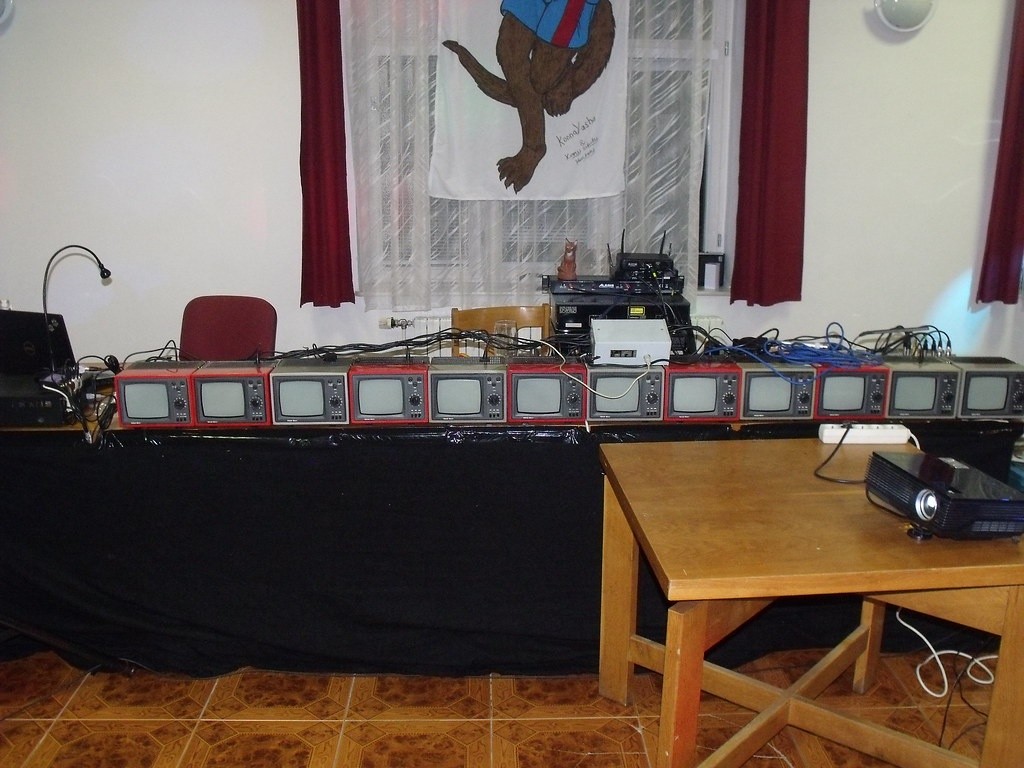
[179,296,278,361]
[450,303,553,358]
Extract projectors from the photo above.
[863,451,1024,540]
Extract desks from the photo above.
[0,349,1024,684]
[598,441,1024,768]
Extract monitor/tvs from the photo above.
[942,357,1024,419]
[815,362,891,422]
[663,362,743,424]
[348,356,430,426]
[883,357,963,420]
[506,362,589,424]
[113,361,205,430]
[586,365,665,424]
[736,360,817,422]
[190,359,279,432]
[426,363,507,424]
[269,357,354,428]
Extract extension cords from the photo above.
[872,348,939,356]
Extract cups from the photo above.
[493,319,519,356]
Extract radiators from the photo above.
[377,317,729,364]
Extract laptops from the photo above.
[0,309,116,387]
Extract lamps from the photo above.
[874,0,938,33]
